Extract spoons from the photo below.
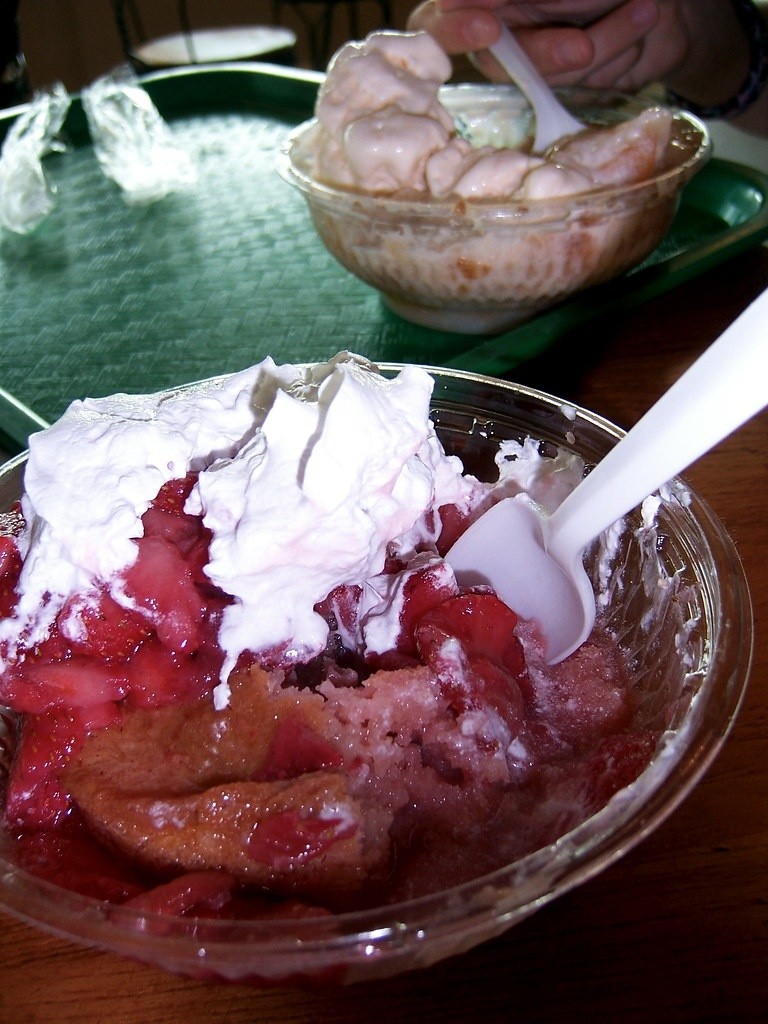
[443,288,768,667]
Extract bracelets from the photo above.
[665,0,768,119]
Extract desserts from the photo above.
[299,32,677,314]
[0,346,646,984]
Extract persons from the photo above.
[405,0,768,138]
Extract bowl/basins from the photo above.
[274,81,714,338]
[0,361,756,987]
